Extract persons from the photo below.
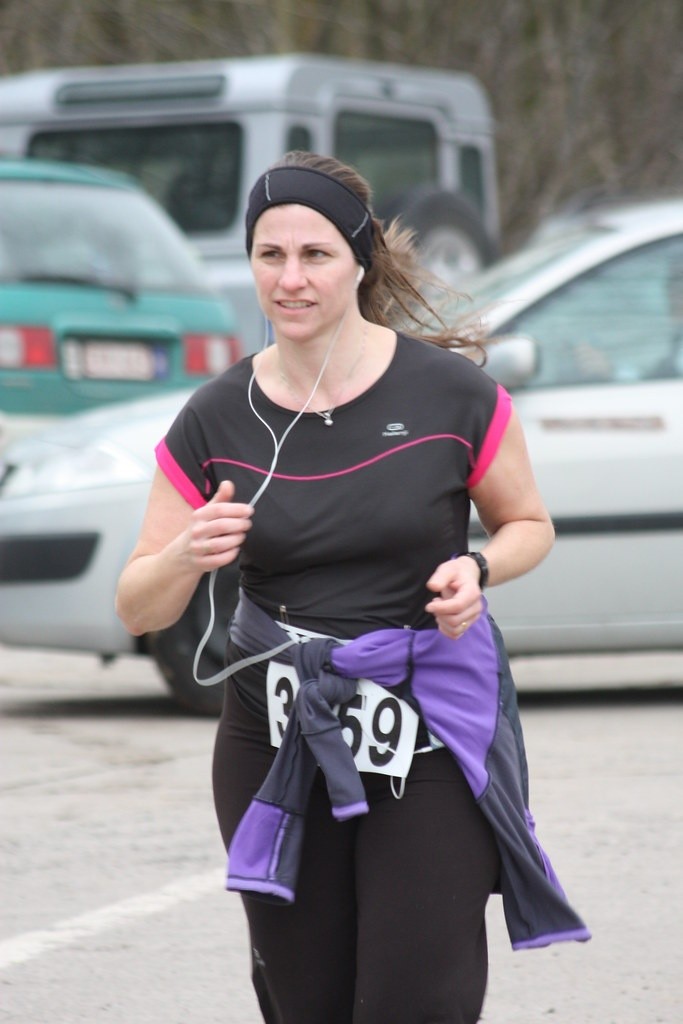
[116,150,556,1024]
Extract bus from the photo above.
[0,198,683,716]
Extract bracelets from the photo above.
[458,548,489,593]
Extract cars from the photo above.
[0,157,241,420]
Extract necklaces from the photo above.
[281,364,359,425]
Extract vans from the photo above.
[4,49,494,356]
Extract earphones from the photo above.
[355,265,364,289]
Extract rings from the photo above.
[461,622,471,633]
